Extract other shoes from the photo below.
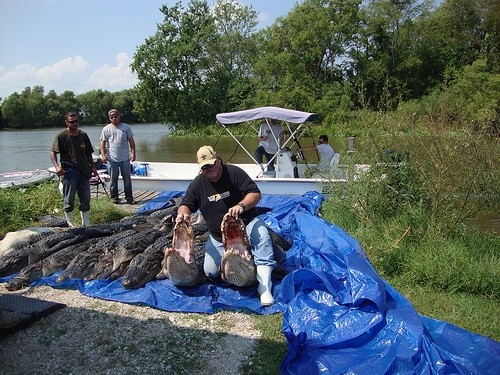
[111,196,120,203]
[127,199,137,205]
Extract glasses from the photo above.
[317,141,324,144]
[201,160,217,170]
[110,115,118,119]
[68,119,80,124]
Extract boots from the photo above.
[79,208,91,226]
[255,265,275,307]
[258,162,265,178]
[64,211,79,228]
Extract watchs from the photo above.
[237,203,246,211]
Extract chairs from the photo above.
[90,169,110,199]
[327,152,340,175]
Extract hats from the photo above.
[108,109,119,117]
[195,145,217,169]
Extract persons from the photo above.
[100,109,137,205]
[50,112,94,228]
[254,119,282,171]
[296,135,335,169]
[175,145,274,306]
